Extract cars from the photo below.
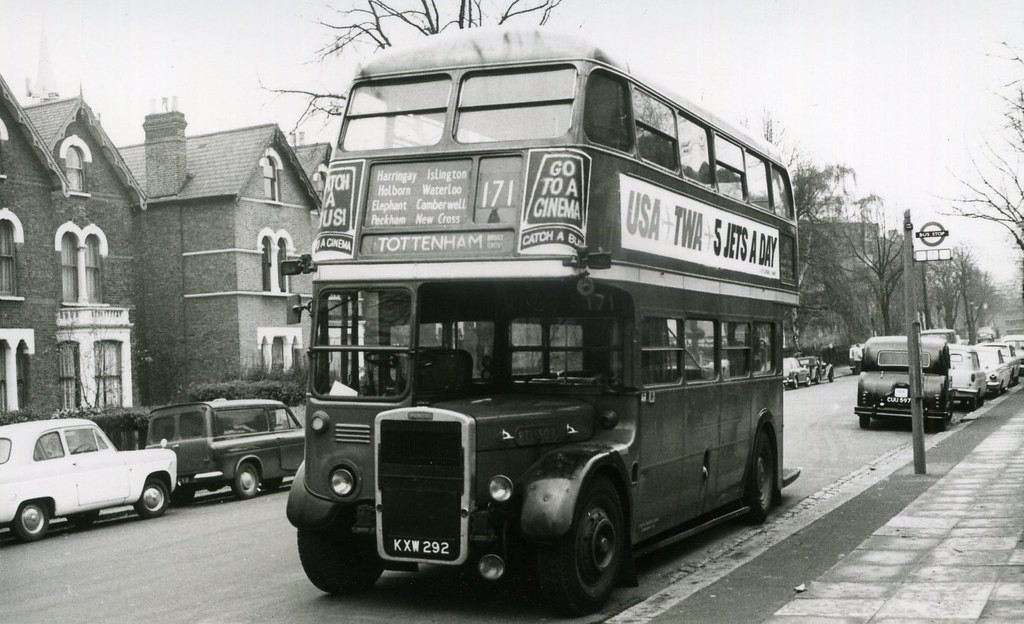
[0,417,179,543]
[144,397,305,508]
[781,328,1024,412]
[853,337,958,432]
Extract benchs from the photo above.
[514,368,708,382]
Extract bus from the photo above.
[279,22,804,620]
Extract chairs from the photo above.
[413,347,474,397]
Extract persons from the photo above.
[854,343,862,375]
[826,342,838,365]
